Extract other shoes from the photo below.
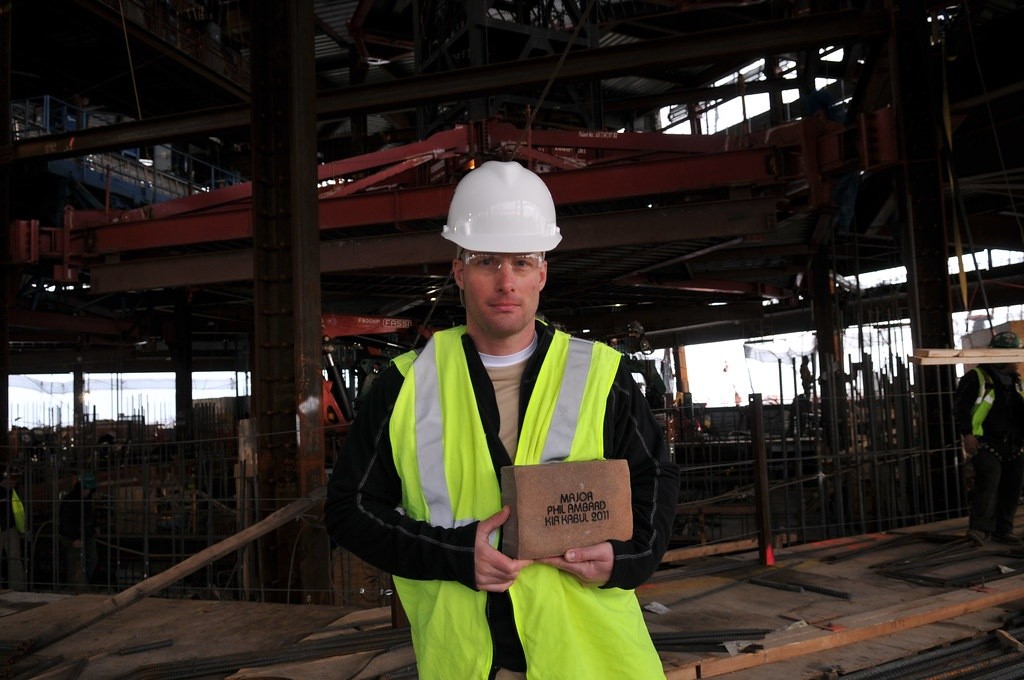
[966,529,1021,546]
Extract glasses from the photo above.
[460,252,544,274]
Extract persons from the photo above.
[954,331,1024,545]
[801,357,812,400]
[325,161,679,680]
[0,473,98,594]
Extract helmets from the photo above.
[990,330,1021,348]
[441,161,563,253]
